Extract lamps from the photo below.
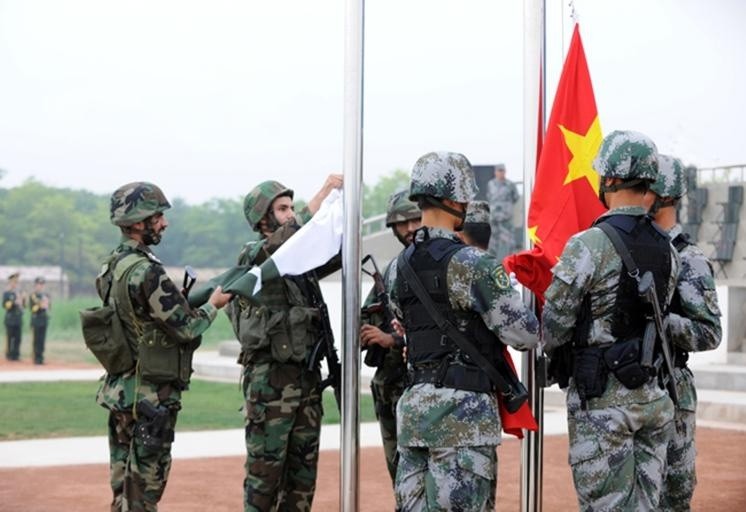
[682,182,745,280]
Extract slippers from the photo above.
[78,300,135,378]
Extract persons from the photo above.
[2,272,28,360]
[29,277,52,364]
[93,182,232,512]
[484,165,519,266]
[387,151,540,512]
[359,189,424,491]
[540,129,676,512]
[222,173,344,512]
[649,153,723,512]
[390,199,492,367]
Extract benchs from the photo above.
[492,21,611,439]
[181,184,346,310]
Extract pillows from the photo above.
[108,180,174,228]
[649,152,689,199]
[242,178,295,233]
[462,200,492,224]
[408,150,480,205]
[590,128,660,185]
[384,186,422,228]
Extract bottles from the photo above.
[35,276,46,285]
[7,271,20,280]
[494,163,505,171]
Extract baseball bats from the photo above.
[361,255,411,391]
[178,267,196,298]
[137,400,169,437]
[638,270,679,404]
[303,274,342,412]
